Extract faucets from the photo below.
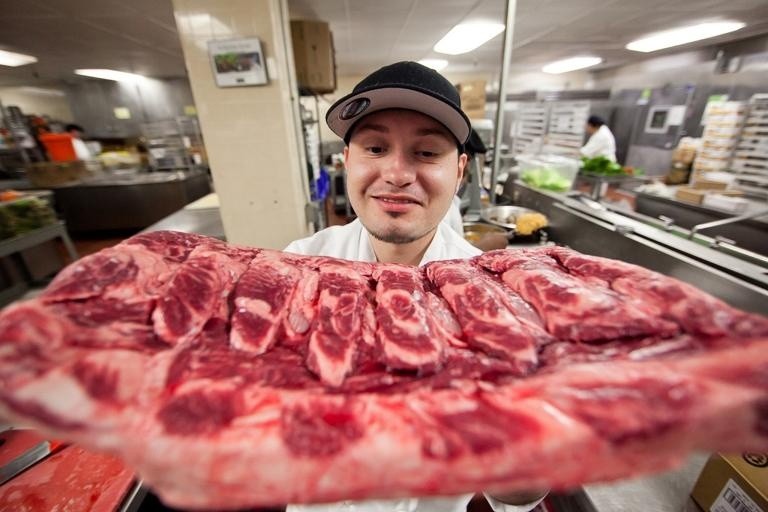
[689,209,768,238]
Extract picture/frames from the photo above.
[206,37,269,89]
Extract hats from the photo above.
[588,116,604,127]
[464,130,487,154]
[326,61,470,147]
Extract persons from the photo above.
[62,123,93,162]
[281,60,554,511]
[443,128,510,254]
[570,115,618,200]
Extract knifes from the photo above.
[0,433,68,486]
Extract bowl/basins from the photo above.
[480,205,544,230]
